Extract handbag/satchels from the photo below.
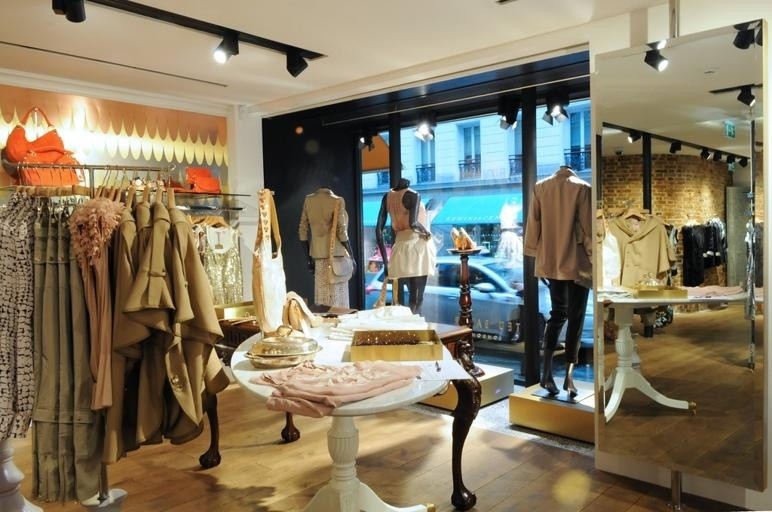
[254,242,287,333]
[284,289,315,331]
[17,147,85,186]
[4,106,64,162]
[193,176,221,193]
[328,259,354,285]
[184,167,212,184]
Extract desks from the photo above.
[229,320,450,512]
[596,284,762,423]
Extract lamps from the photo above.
[51,0,327,77]
[357,83,570,146]
[644,41,669,71]
[627,131,748,167]
[733,19,762,50]
[711,83,762,106]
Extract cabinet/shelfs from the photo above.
[445,248,485,377]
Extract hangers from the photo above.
[188,206,228,228]
[8,161,178,206]
[597,196,670,225]
[683,213,720,225]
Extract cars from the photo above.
[365,256,593,359]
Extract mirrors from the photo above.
[593,17,771,510]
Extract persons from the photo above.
[297,185,358,314]
[518,165,592,397]
[374,176,433,314]
